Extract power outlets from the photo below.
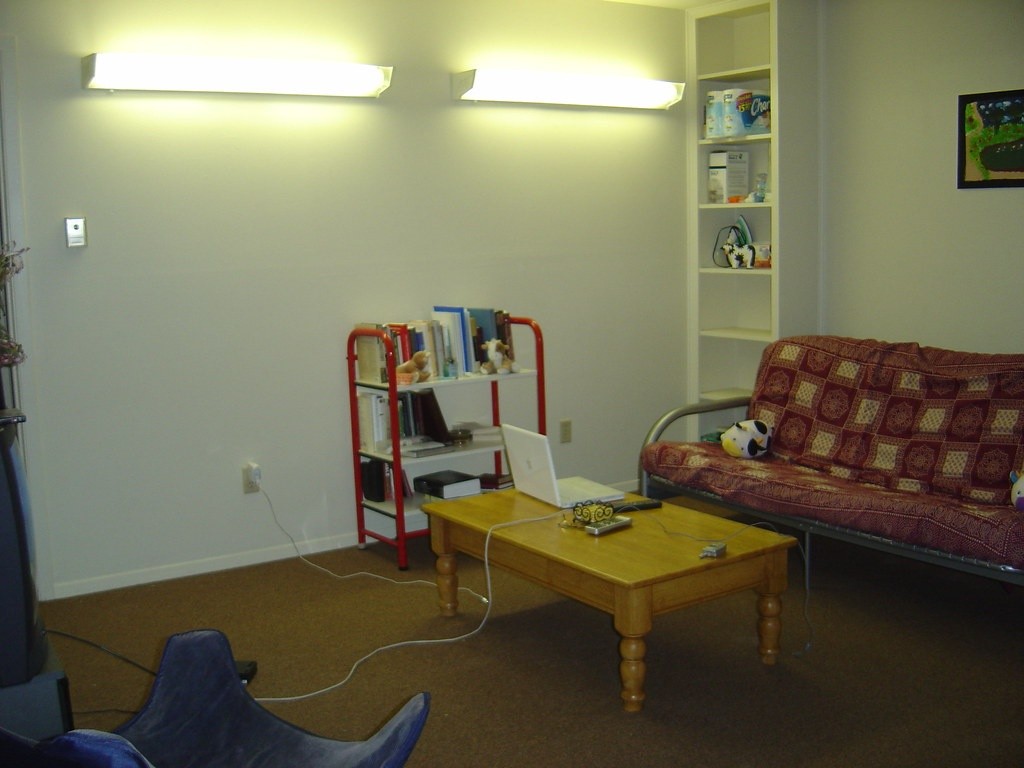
[242,466,260,493]
[560,420,571,443]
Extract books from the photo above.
[353,305,516,385]
[355,459,515,503]
[357,388,502,459]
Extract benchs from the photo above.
[638,335,1024,591]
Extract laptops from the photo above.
[501,423,626,508]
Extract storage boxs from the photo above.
[708,150,749,204]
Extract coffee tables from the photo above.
[420,488,799,714]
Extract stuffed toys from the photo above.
[720,418,771,459]
[1009,468,1024,511]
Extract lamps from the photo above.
[82,53,393,98]
[451,70,684,109]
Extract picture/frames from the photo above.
[956,89,1024,190]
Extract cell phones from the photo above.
[699,542,726,559]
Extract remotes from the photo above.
[584,514,632,535]
[610,498,662,510]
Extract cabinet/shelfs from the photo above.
[346,317,548,572]
[685,0,820,444]
